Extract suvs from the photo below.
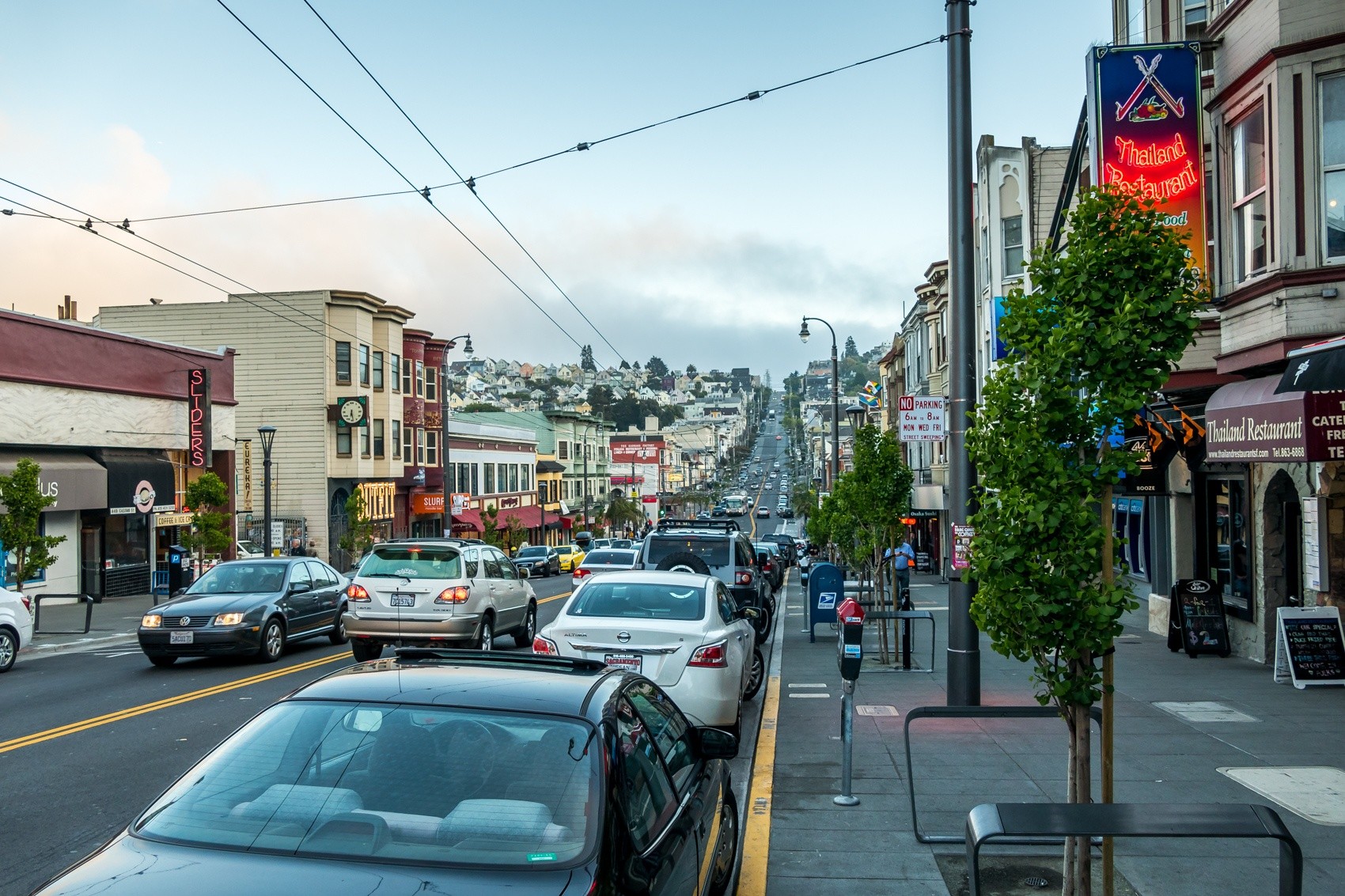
[570,531,600,553]
[634,518,776,644]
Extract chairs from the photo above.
[258,571,284,592]
[503,725,590,836]
[341,723,461,819]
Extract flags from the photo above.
[1165,398,1207,474]
[1133,413,1181,477]
[1142,404,1187,458]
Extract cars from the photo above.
[512,545,561,577]
[611,539,633,549]
[137,555,351,667]
[749,533,804,590]
[695,388,793,523]
[237,539,287,559]
[594,538,613,549]
[630,542,642,550]
[572,549,640,593]
[31,649,739,895]
[552,544,585,573]
[341,537,538,663]
[533,570,764,753]
[0,586,33,672]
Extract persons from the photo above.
[306,541,317,557]
[290,539,307,556]
[910,531,919,571]
[884,536,915,600]
[885,547,892,585]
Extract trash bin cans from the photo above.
[809,562,844,644]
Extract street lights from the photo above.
[441,333,474,537]
[539,484,547,544]
[258,426,277,557]
[799,314,839,490]
[585,423,603,531]
[632,449,647,509]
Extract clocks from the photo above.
[341,399,363,424]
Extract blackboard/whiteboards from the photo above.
[1167,578,1232,659]
[1273,606,1345,690]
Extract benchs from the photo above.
[230,782,576,846]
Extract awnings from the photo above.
[451,503,562,532]
[0,447,176,516]
[1203,334,1344,464]
